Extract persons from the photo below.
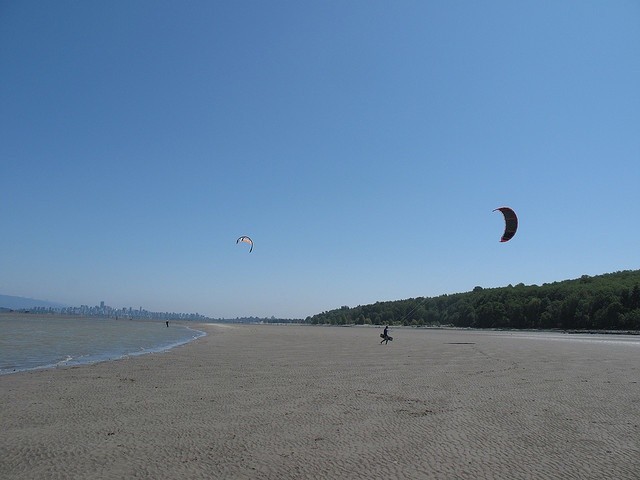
[381,325,389,344]
[165,320,169,327]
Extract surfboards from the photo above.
[380,334,393,341]
